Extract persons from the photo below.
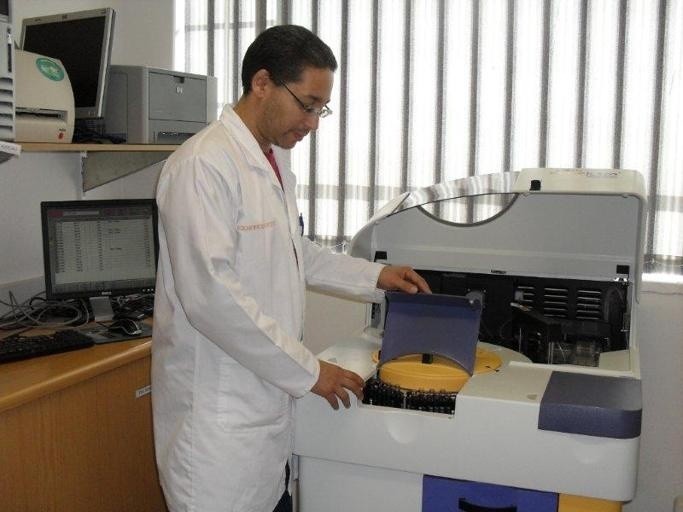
[148,23,436,512]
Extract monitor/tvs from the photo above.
[40,199,159,322]
[19,7,116,119]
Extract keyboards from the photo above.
[0,329,94,364]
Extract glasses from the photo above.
[272,73,332,118]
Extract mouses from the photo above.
[108,318,142,336]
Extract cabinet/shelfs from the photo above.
[3,344,166,511]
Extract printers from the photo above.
[86,64,218,145]
